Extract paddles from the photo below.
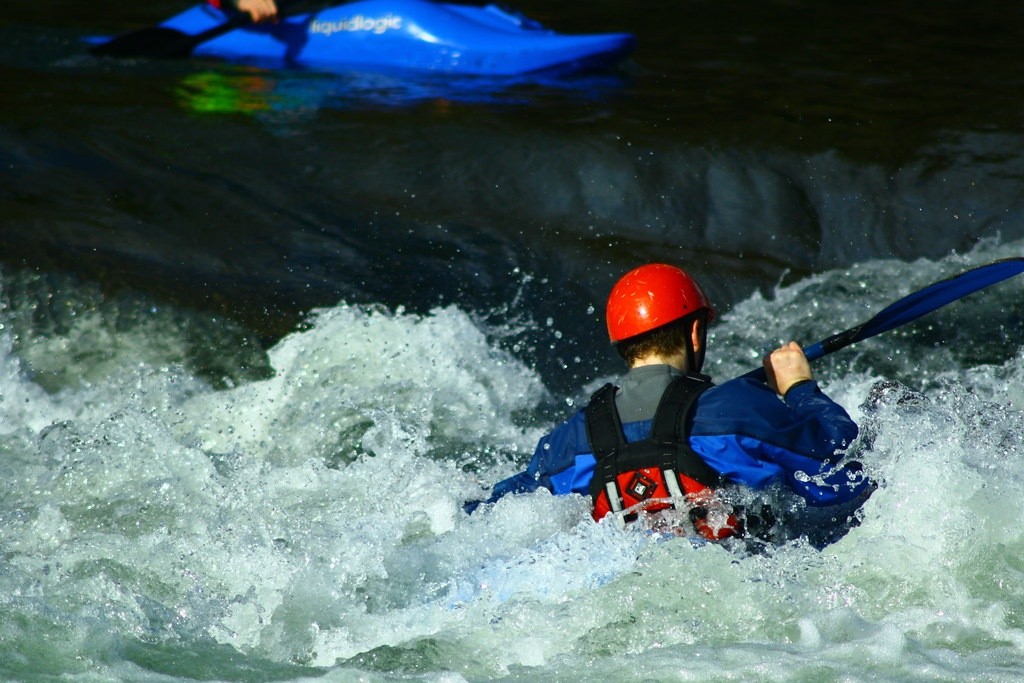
[467,257,1024,512]
[109,2,290,64]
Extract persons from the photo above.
[528,263,883,556]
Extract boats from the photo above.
[81,0,632,77]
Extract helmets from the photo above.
[605,264,717,342]
